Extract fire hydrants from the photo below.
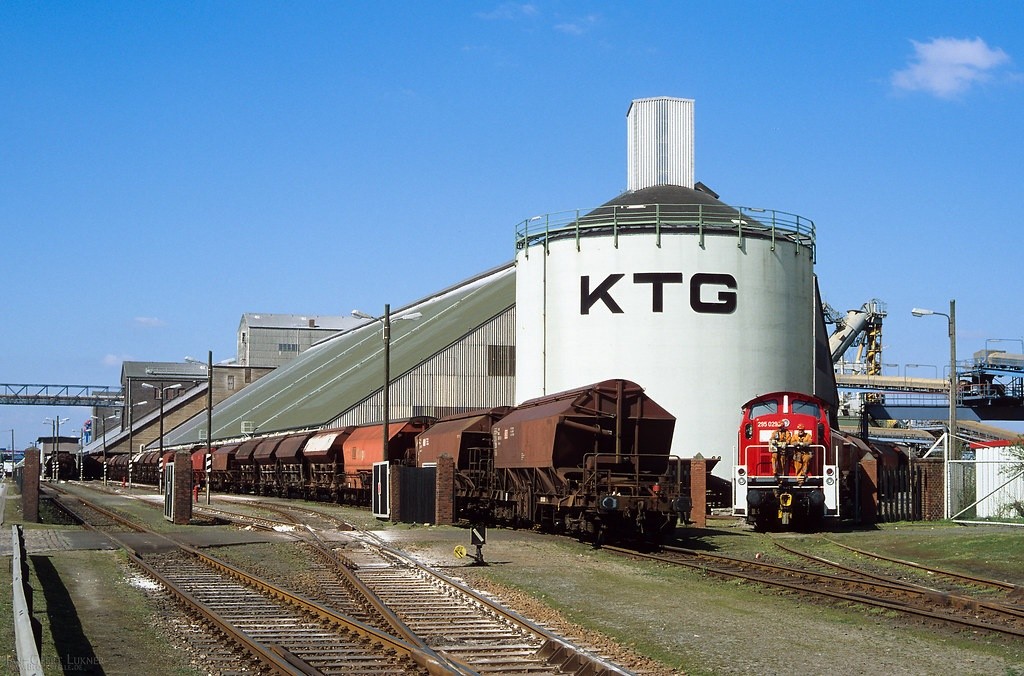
[193,486,199,503]
[122,477,126,489]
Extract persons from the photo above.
[770,422,791,486]
[793,424,812,487]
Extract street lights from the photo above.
[115,399,147,490]
[910,299,956,459]
[185,350,237,505]
[91,415,116,486]
[42,415,70,484]
[351,303,423,462]
[72,428,91,482]
[142,382,182,497]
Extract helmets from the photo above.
[796,423,805,430]
[777,421,784,428]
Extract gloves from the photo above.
[794,442,799,447]
[782,444,786,449]
[772,440,776,446]
[803,442,809,447]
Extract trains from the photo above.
[732,391,919,530]
[79,378,697,548]
